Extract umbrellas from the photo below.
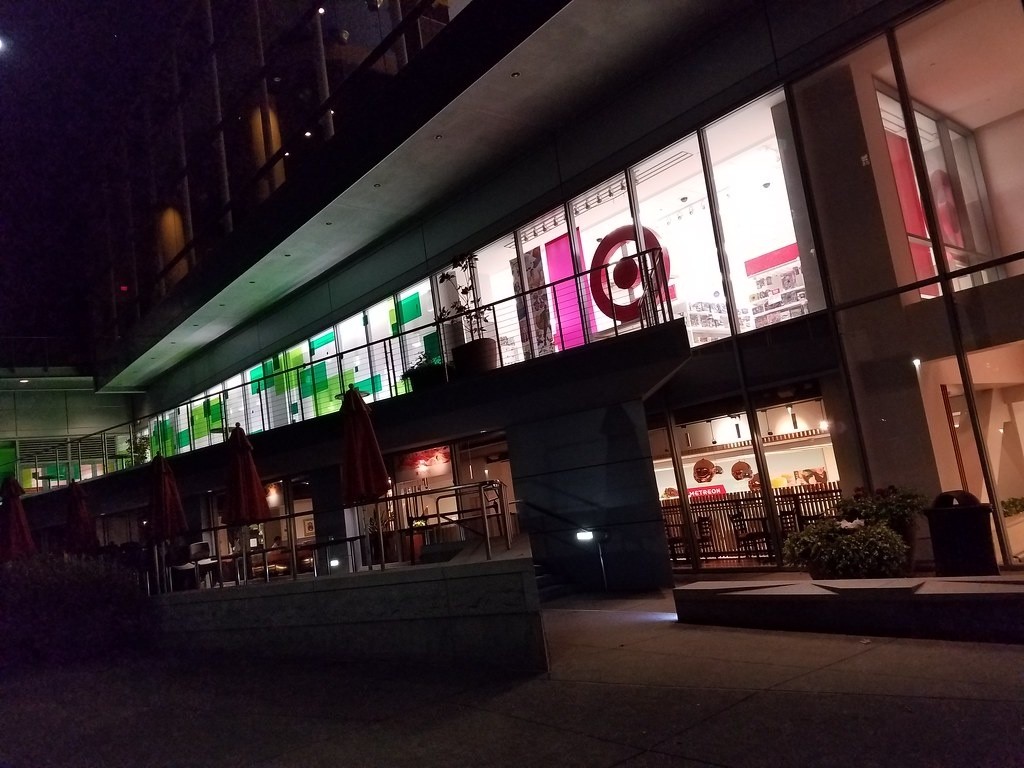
[339,383,393,572]
[60,478,100,557]
[221,422,271,585]
[0,475,39,564]
[145,451,189,593]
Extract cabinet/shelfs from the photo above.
[666,300,692,348]
[748,257,809,330]
[687,300,753,348]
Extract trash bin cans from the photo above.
[922,488,999,576]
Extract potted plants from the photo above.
[432,255,497,377]
[400,352,452,391]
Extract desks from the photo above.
[35,475,64,490]
[108,453,138,470]
[212,534,369,586]
[335,390,370,401]
[210,427,236,441]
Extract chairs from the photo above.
[802,512,825,528]
[778,509,798,542]
[662,518,691,567]
[729,511,769,559]
[695,516,719,561]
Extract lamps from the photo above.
[519,170,641,243]
[662,398,826,453]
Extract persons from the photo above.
[271,535,283,553]
